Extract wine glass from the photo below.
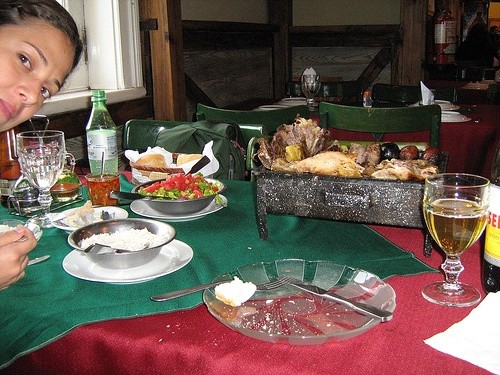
[303,74,321,112]
[16,130,66,229]
[421,172,491,308]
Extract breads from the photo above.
[176,153,203,165]
[214,276,257,307]
[134,154,169,167]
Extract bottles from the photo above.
[86,89,119,175]
[481,141,500,294]
[466,10,485,33]
[363,91,372,108]
[434,10,457,55]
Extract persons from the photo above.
[0,0,84,293]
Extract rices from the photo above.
[80,226,162,250]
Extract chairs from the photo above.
[123,60,500,183]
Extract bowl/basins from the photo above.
[67,218,177,269]
[131,176,227,215]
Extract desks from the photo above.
[309,104,500,183]
[0,169,500,375]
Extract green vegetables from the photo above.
[139,172,223,205]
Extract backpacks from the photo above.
[150,117,248,182]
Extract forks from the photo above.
[150,276,296,301]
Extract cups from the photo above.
[85,170,121,207]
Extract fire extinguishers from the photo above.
[433,0,457,64]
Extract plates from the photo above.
[414,99,472,122]
[203,258,397,345]
[62,239,194,286]
[51,206,129,235]
[130,193,228,222]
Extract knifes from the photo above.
[277,275,394,323]
[26,254,51,266]
